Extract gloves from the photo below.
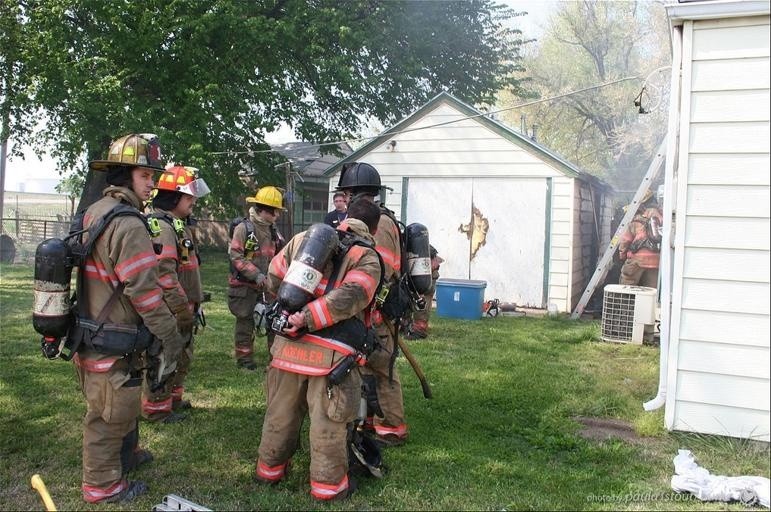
[176,308,196,330]
[255,272,269,290]
[161,327,192,366]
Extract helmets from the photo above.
[247,185,287,211]
[335,162,392,190]
[152,165,203,199]
[88,133,168,173]
[639,188,653,203]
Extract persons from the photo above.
[254,200,385,503]
[336,162,409,448]
[228,185,288,370]
[618,187,663,288]
[141,167,210,425]
[73,135,185,505]
[325,193,347,228]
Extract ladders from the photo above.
[152,493,214,512]
[571,132,667,321]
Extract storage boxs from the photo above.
[436,278,486,321]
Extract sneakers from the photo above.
[399,324,427,341]
[121,445,152,472]
[170,399,192,410]
[236,358,258,371]
[91,480,148,502]
[141,411,188,424]
[330,478,361,502]
